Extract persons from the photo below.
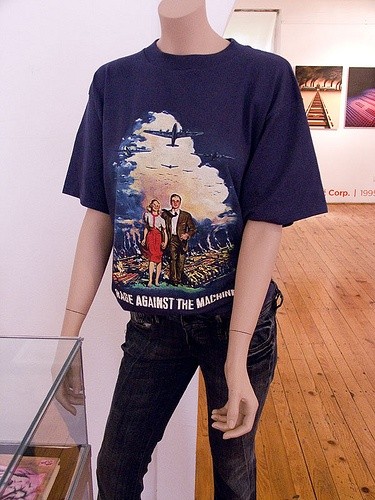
[51,1,329,500]
[161,193,196,286]
[141,200,169,287]
